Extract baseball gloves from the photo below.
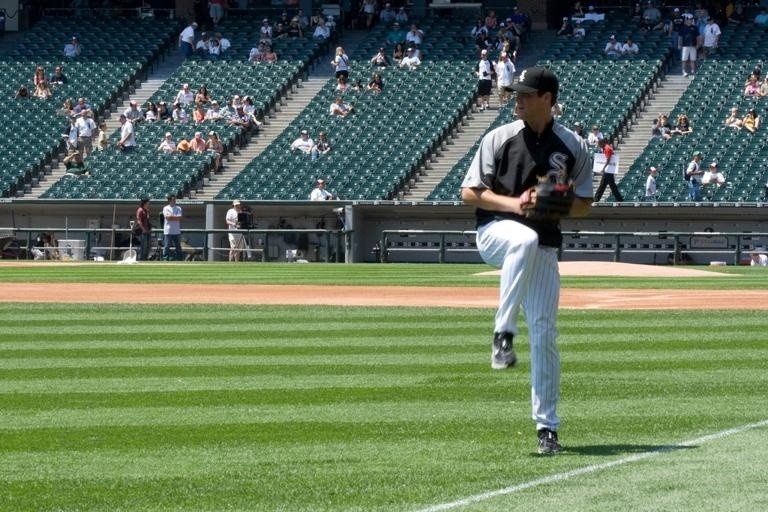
[520,176,574,222]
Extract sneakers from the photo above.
[491,331,516,370]
[537,428,561,454]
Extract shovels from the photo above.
[123,233,137,261]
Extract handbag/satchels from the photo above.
[131,223,142,236]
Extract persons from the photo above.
[208,0,223,25]
[179,23,231,61]
[136,195,152,260]
[49,66,68,85]
[33,68,47,85]
[472,6,521,112]
[551,102,564,117]
[645,167,660,198]
[311,130,333,161]
[15,85,30,98]
[64,37,81,57]
[329,48,384,118]
[138,3,154,19]
[557,0,768,77]
[61,81,262,178]
[685,150,704,201]
[33,80,51,97]
[652,112,693,137]
[361,1,425,72]
[162,195,185,260]
[249,11,336,64]
[224,199,247,262]
[575,122,603,147]
[458,70,595,454]
[309,178,333,202]
[291,130,314,153]
[593,140,624,201]
[667,242,694,263]
[724,66,768,134]
[749,250,768,267]
[699,162,725,190]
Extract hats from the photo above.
[502,67,557,96]
[233,200,241,206]
